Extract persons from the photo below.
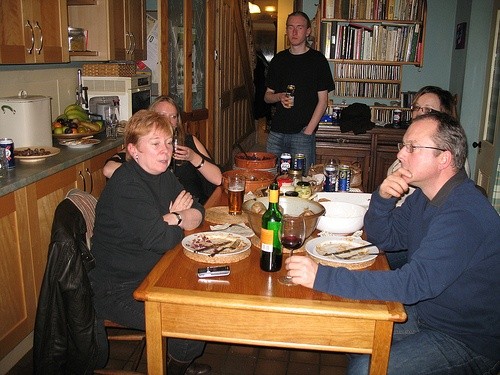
[383,86,455,271]
[265,11,335,168]
[92,110,212,375]
[103,95,222,207]
[285,112,500,375]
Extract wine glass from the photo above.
[277,218,307,286]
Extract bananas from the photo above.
[64,105,88,121]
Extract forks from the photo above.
[210,238,241,258]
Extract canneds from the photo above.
[337,165,351,192]
[393,110,401,128]
[280,153,292,175]
[0,138,15,170]
[323,166,335,192]
[276,168,311,199]
[293,153,305,173]
[331,107,341,126]
[285,85,295,106]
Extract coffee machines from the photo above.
[89,96,120,140]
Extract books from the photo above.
[319,0,423,126]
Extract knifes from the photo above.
[322,244,377,256]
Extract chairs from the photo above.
[33,191,145,375]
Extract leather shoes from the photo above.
[166,354,212,375]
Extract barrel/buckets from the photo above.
[0,90,53,147]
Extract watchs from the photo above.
[195,158,205,169]
[172,212,182,226]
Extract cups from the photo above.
[228,174,246,215]
[0,148,8,179]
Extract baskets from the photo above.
[83,63,137,77]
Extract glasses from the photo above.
[397,143,447,153]
[410,105,432,113]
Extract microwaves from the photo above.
[81,74,151,123]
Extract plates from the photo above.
[59,139,101,149]
[181,232,252,264]
[306,237,379,270]
[14,147,60,162]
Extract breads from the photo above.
[252,202,314,217]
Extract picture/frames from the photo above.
[454,20,469,52]
[285,34,290,49]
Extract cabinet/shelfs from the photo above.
[315,126,411,192]
[318,0,427,100]
[0,144,124,375]
[0,0,147,64]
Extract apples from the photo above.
[52,115,102,134]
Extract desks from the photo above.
[133,184,407,375]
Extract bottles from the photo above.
[259,181,283,272]
[67,27,85,51]
[278,170,323,199]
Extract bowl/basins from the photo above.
[234,152,278,173]
[315,200,366,235]
[223,169,276,199]
[242,198,325,246]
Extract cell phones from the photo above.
[197,264,230,278]
